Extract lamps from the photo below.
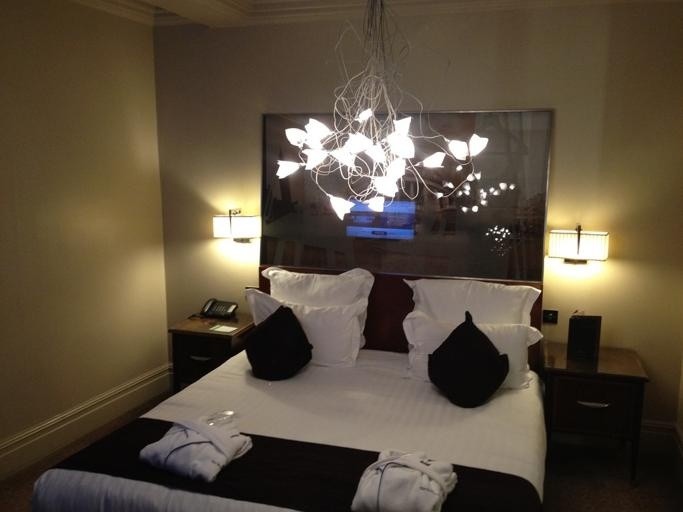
[548,225,609,265]
[276,0,489,221]
[212,209,263,243]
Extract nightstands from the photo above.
[544,341,649,487]
[168,311,255,396]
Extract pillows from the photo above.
[280,294,368,369]
[401,277,542,326]
[427,311,509,408]
[241,304,312,382]
[261,268,375,306]
[403,310,543,390]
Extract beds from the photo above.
[30,268,547,512]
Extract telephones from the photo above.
[201,299,238,318]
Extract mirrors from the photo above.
[260,108,555,286]
